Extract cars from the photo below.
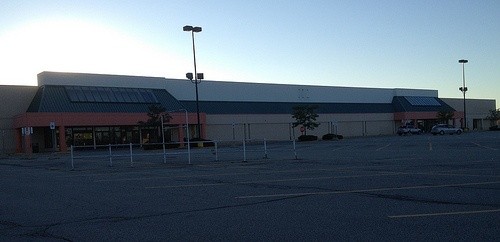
[431,123,463,135]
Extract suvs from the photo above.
[397,125,422,136]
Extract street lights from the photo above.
[183,24,204,139]
[459,58,469,129]
